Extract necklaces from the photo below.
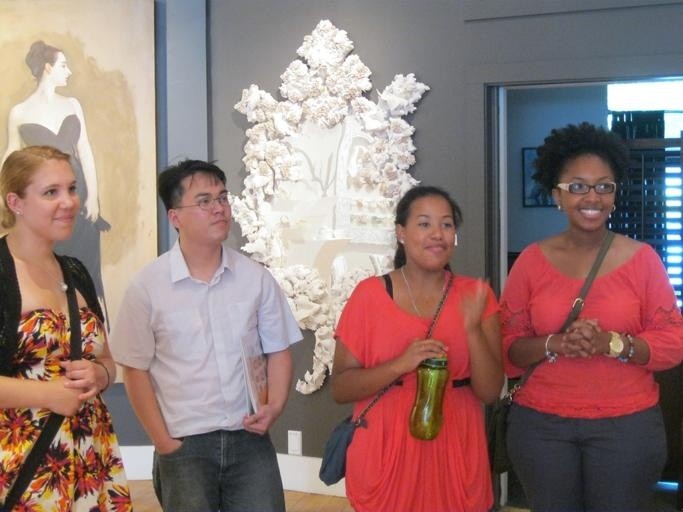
[27,255,69,294]
[401,265,422,318]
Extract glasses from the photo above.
[552,181,619,195]
[172,193,237,212]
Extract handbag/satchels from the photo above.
[317,411,369,488]
[484,389,517,477]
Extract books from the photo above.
[239,327,268,416]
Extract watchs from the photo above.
[603,330,624,359]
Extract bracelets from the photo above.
[93,361,110,392]
[545,334,559,359]
[618,333,634,363]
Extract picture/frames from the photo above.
[521,146,559,209]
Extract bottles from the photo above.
[410,355,448,442]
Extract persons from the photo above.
[331,186,498,512]
[122,160,294,512]
[498,122,683,512]
[0,145,117,512]
[1,40,111,337]
